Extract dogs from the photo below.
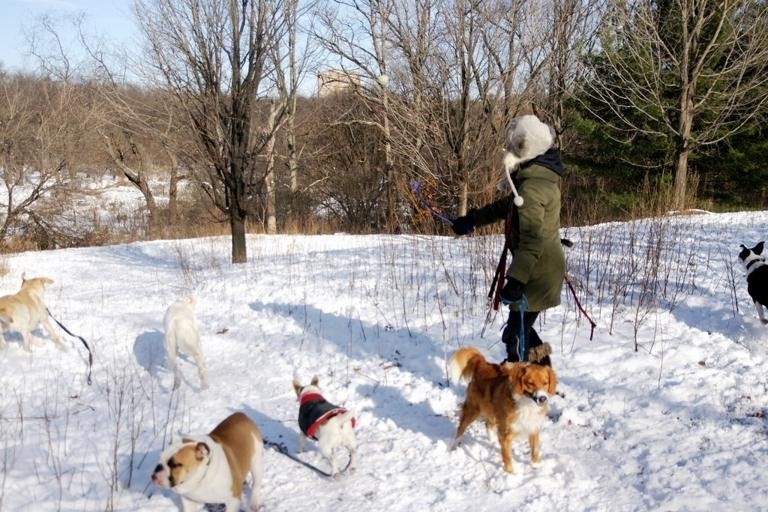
[293,375,358,481]
[163,295,210,390]
[0,272,64,353]
[447,347,556,473]
[151,412,263,512]
[738,241,768,326]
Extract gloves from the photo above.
[452,216,473,235]
[498,278,524,305]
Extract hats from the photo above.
[502,115,556,169]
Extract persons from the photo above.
[451,115,565,367]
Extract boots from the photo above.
[500,341,552,367]
